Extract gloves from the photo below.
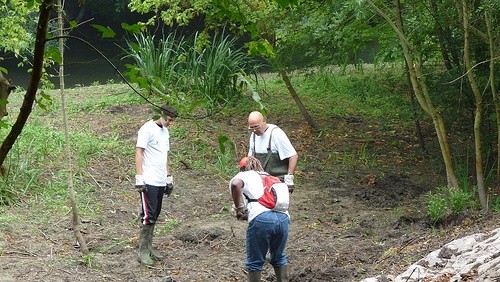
[236,208,249,220]
[164,175,174,198]
[135,175,148,193]
[284,175,295,194]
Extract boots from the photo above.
[248,271,261,282]
[274,266,289,282]
[138,223,154,267]
[148,225,165,261]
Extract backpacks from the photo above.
[243,169,289,211]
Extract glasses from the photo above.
[248,122,263,131]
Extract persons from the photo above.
[229,156,291,282]
[135,103,178,266]
[248,111,299,194]
